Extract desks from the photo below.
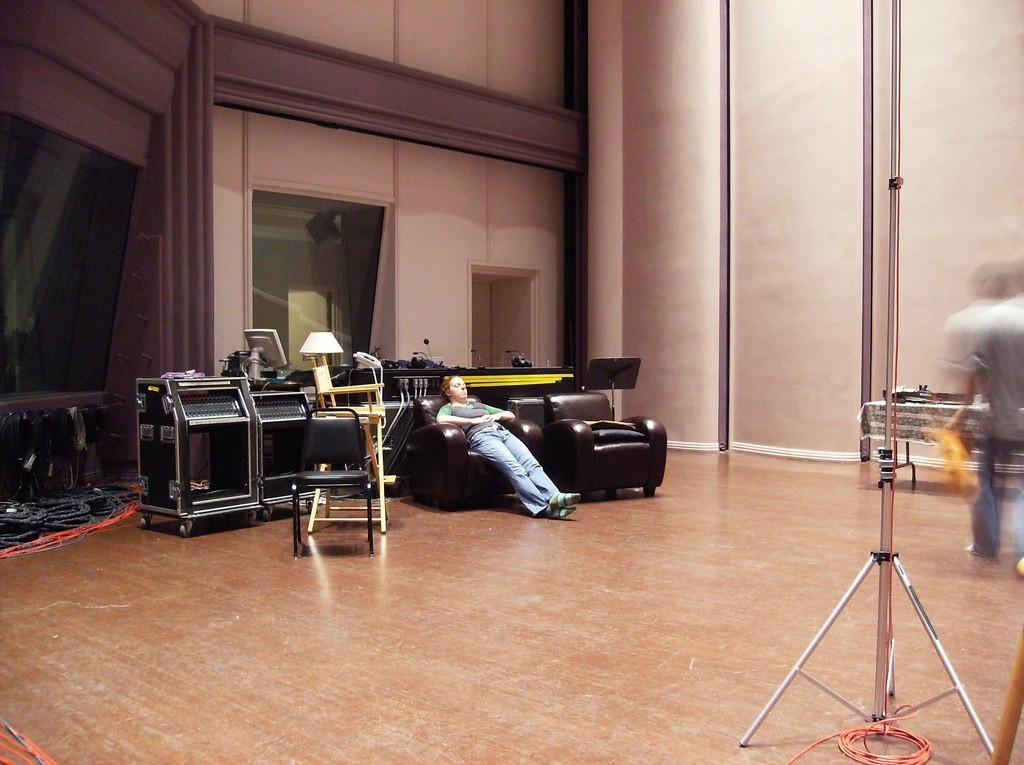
[858,401,1023,491]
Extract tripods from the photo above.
[740,0,996,758]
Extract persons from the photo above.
[944,258,1024,559]
[435,375,581,518]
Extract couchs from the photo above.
[542,394,667,500]
[406,394,542,508]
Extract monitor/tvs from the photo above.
[244,328,287,380]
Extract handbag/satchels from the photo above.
[937,426,978,500]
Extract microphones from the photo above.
[413,339,434,367]
[506,349,527,362]
[470,350,485,369]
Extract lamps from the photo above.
[299,331,344,354]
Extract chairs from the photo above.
[292,408,373,558]
